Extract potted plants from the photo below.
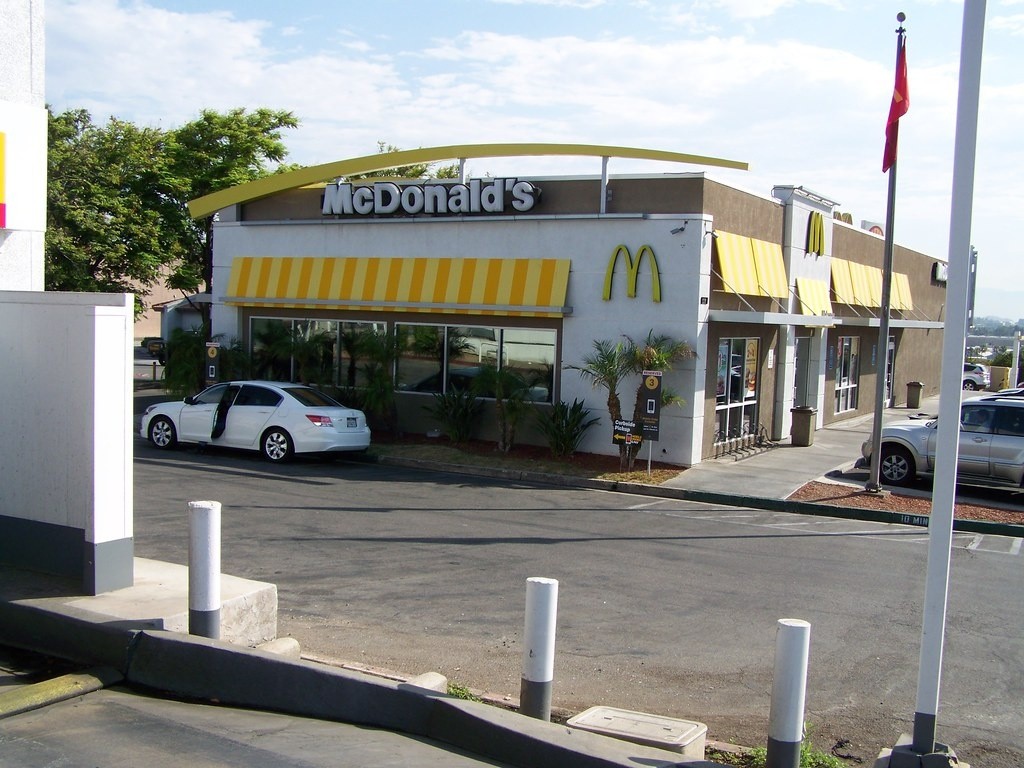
[989,351,1019,391]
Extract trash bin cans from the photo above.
[789,405,818,447]
[906,381,925,409]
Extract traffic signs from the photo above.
[612,419,645,445]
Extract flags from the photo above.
[881,35,910,172]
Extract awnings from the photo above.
[221,255,572,320]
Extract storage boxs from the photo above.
[567,705,708,761]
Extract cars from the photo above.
[139,380,372,462]
[962,362,991,391]
[401,366,530,402]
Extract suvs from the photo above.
[862,388,1024,494]
